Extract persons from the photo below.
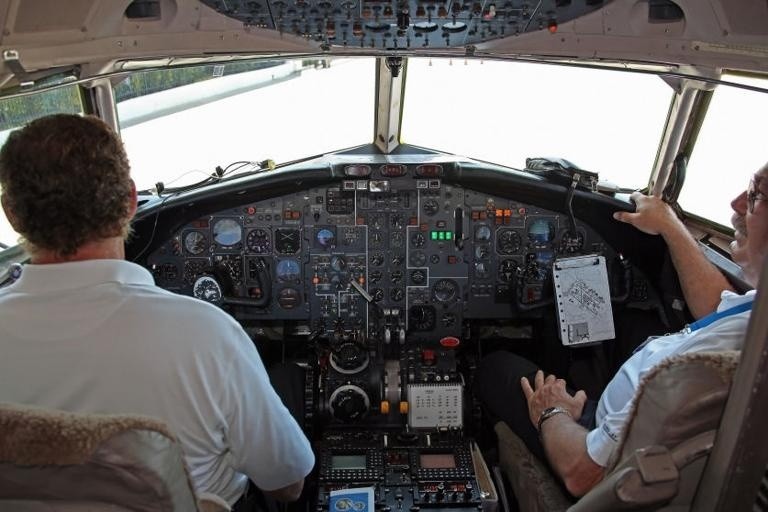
[0,112,316,512]
[481,159,768,501]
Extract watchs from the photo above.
[537,406,573,432]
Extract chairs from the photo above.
[494,349,768,511]
[0,402,234,512]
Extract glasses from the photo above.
[748,180,767,213]
